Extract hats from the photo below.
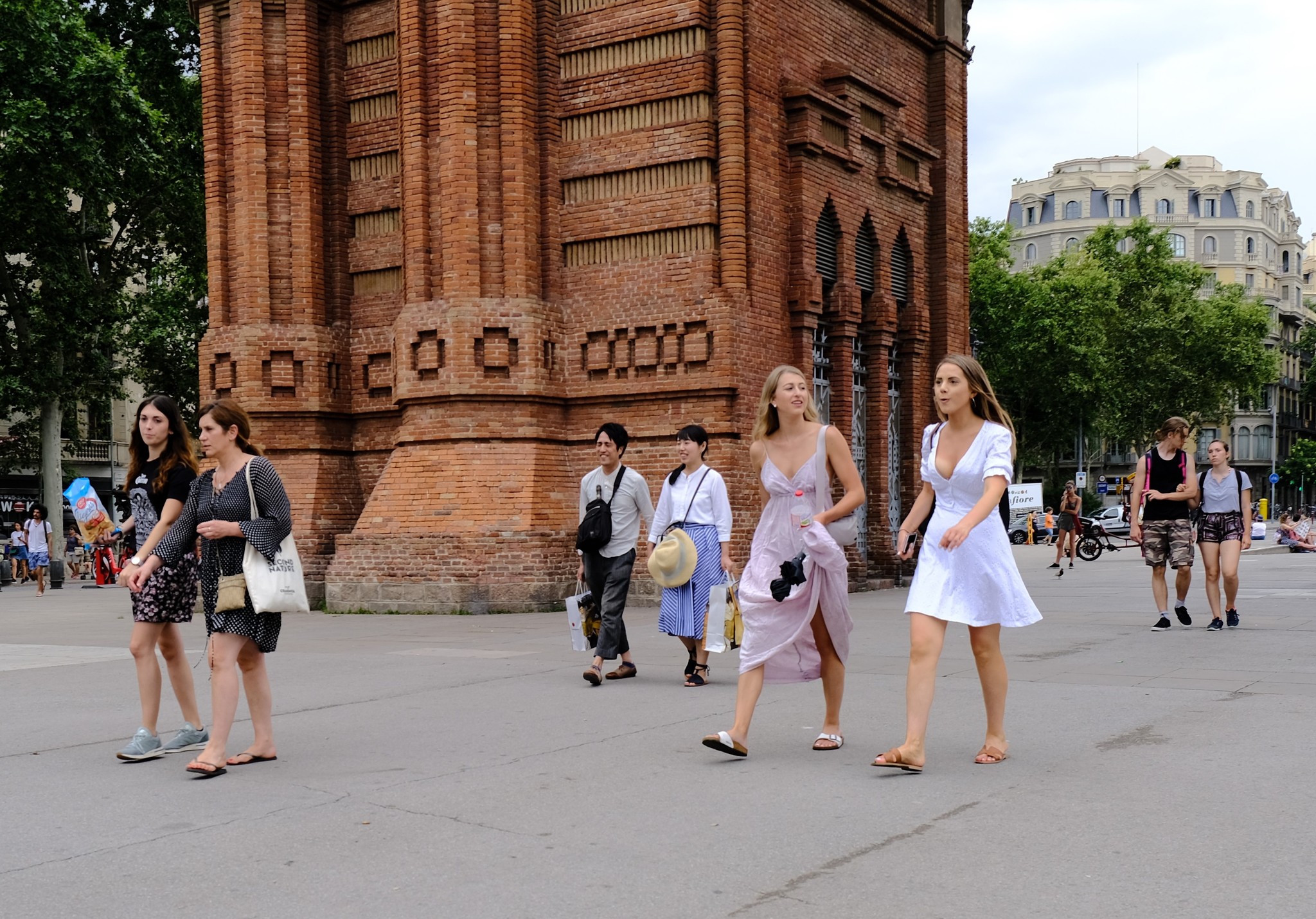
[647,528,697,587]
[1255,515,1264,520]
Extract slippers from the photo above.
[813,733,845,750]
[870,748,923,771]
[36,580,47,597]
[702,731,748,757]
[974,745,1007,763]
[186,752,278,775]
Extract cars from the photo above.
[1007,512,1060,545]
[1082,504,1144,538]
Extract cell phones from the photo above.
[903,533,918,553]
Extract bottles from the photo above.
[790,490,812,563]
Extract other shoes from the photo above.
[1042,539,1054,546]
[71,571,79,579]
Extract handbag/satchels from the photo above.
[74,546,84,556]
[701,568,744,652]
[576,463,625,552]
[214,573,248,613]
[242,456,311,615]
[1073,514,1082,535]
[10,547,19,556]
[825,511,858,546]
[565,578,602,651]
[1272,532,1282,542]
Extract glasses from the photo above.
[1064,486,1073,489]
[68,528,73,531]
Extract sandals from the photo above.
[684,647,710,686]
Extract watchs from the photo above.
[130,557,144,567]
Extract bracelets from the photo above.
[115,526,124,540]
[899,529,910,533]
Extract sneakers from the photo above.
[583,664,637,684]
[1047,562,1074,569]
[117,720,210,759]
[1151,606,1192,632]
[1206,607,1239,631]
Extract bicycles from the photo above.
[1075,514,1146,562]
[1274,508,1293,522]
[91,535,135,582]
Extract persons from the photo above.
[7,503,83,597]
[702,366,867,756]
[1129,416,1198,631]
[94,396,209,759]
[1176,439,1253,632]
[575,422,655,686]
[646,426,734,687]
[871,355,1044,772]
[1031,481,1082,569]
[1251,512,1316,553]
[125,400,291,777]
